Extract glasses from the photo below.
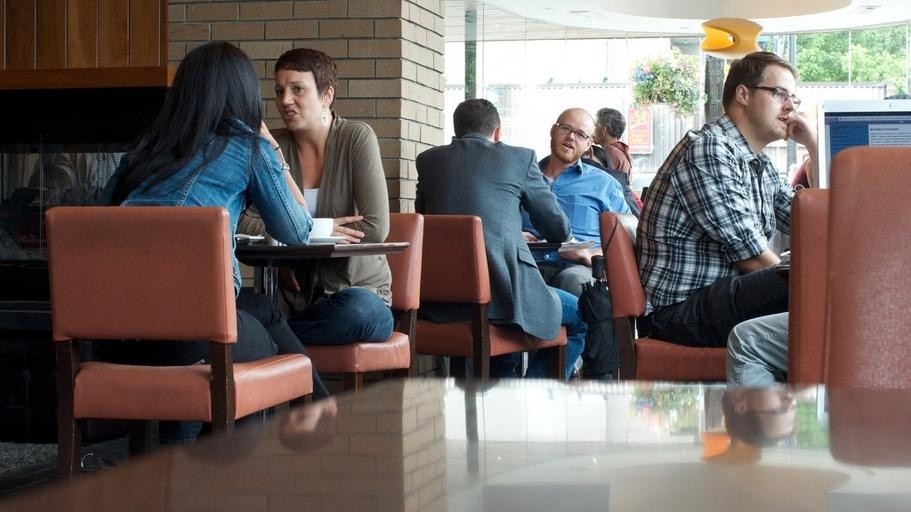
[554,125,591,141]
[746,85,802,106]
[746,406,787,416]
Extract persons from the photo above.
[278,397,338,454]
[790,146,812,191]
[94,39,335,444]
[236,49,393,342]
[633,52,819,385]
[414,98,632,378]
[726,308,790,383]
[721,384,798,448]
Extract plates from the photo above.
[309,236,345,244]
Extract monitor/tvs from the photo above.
[816,99,911,191]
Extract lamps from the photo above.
[699,0,764,59]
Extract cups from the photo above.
[310,218,334,237]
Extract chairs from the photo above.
[788,189,829,384]
[45,206,313,481]
[395,214,568,380]
[599,212,727,380]
[279,213,424,409]
[829,146,911,390]
